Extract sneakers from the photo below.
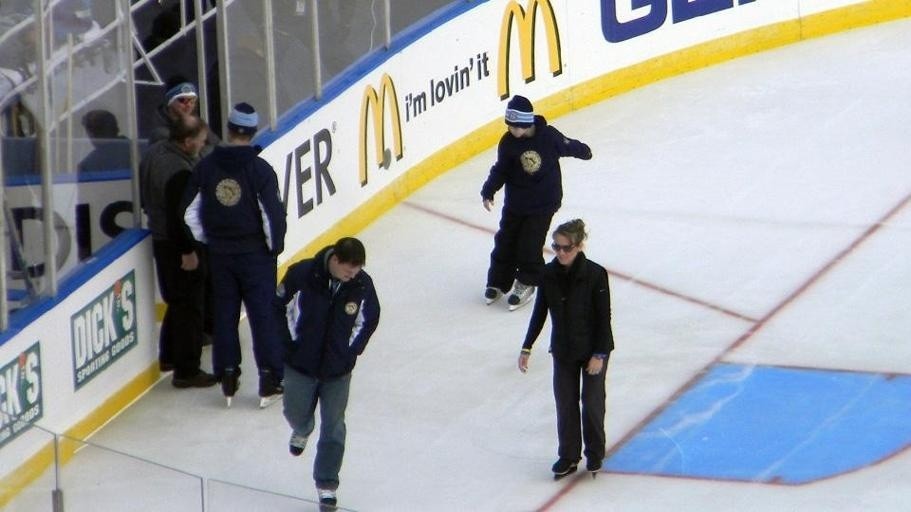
[160,358,284,397]
[484,280,535,305]
[586,458,602,472]
[290,429,308,456]
[318,486,337,505]
[553,458,578,475]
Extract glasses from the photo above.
[176,97,196,105]
[551,242,572,252]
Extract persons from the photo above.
[178,102,287,397]
[78,109,132,177]
[139,114,218,388]
[148,74,222,347]
[480,95,593,306]
[518,217,614,477]
[273,236,381,512]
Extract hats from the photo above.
[227,103,258,135]
[505,95,534,127]
[160,76,198,108]
